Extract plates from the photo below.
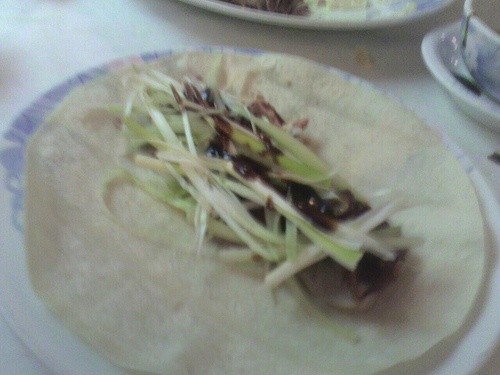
[0,42,499,372]
[173,0,458,30]
[420,18,500,133]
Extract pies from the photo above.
[22,49,488,375]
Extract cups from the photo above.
[457,0,499,104]
